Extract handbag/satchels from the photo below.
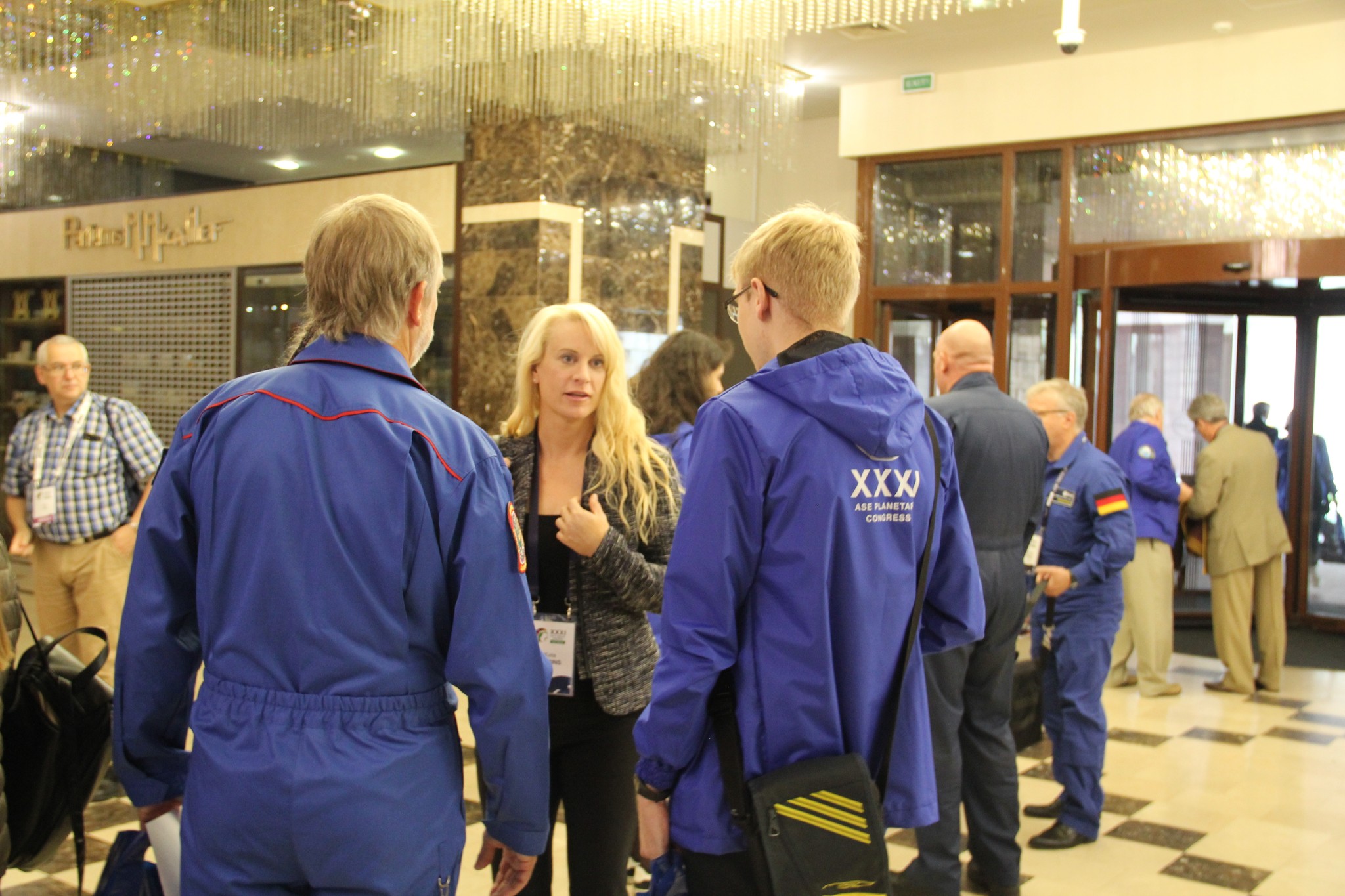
[1319,495,1345,565]
[1009,579,1056,753]
[0,626,114,870]
[748,750,890,896]
[101,829,163,896]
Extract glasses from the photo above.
[44,361,88,373]
[724,280,778,324]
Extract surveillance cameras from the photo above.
[1054,28,1086,53]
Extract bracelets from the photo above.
[1070,570,1079,589]
[633,772,671,803]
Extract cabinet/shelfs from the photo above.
[0,276,68,452]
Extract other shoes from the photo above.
[886,867,962,896]
[956,861,1021,896]
[1108,674,1138,687]
[1144,683,1182,696]
[1254,677,1279,692]
[627,856,651,893]
[1204,681,1242,693]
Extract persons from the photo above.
[0,336,162,804]
[104,194,551,896]
[1183,394,1295,693]
[1024,377,1136,851]
[628,330,735,651]
[887,320,1049,896]
[1243,402,1278,446]
[490,303,685,896]
[632,203,986,896]
[1277,410,1338,607]
[1108,393,1192,696]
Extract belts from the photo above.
[57,510,134,545]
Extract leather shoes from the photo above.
[1023,801,1061,818]
[1029,821,1095,849]
